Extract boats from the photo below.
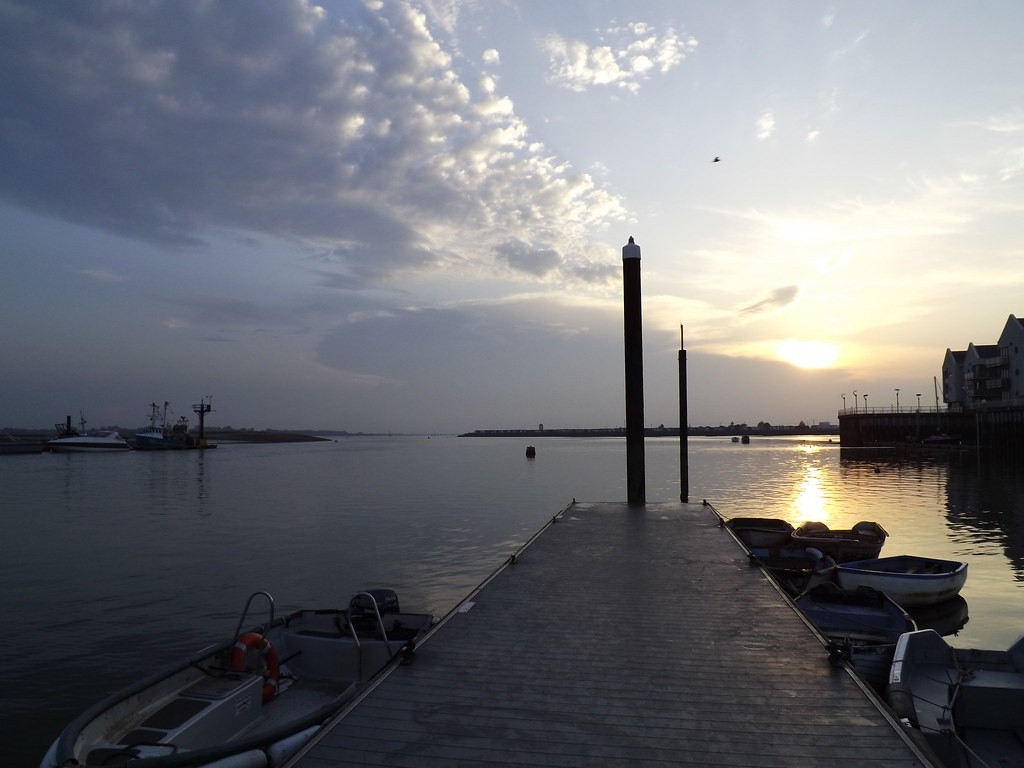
[790,520,890,561]
[793,585,918,681]
[47,430,131,452]
[37,587,443,768]
[134,424,190,448]
[726,515,794,550]
[905,593,971,639]
[886,628,1024,768]
[196,443,218,448]
[835,554,970,603]
[741,435,750,445]
[748,544,837,596]
[731,437,739,442]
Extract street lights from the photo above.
[853,390,858,414]
[916,393,921,409]
[894,388,900,413]
[863,394,868,413]
[841,393,846,409]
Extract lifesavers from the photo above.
[229,632,281,699]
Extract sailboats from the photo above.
[921,375,964,449]
